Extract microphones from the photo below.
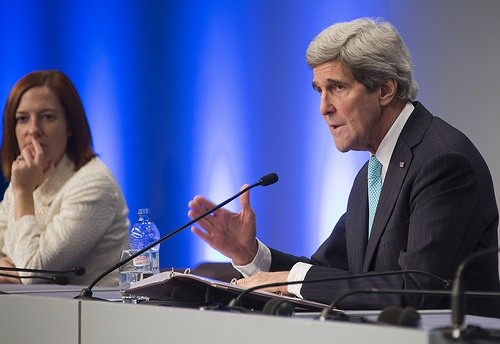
[0,272,69,286]
[74,173,279,301]
[0,267,85,276]
[199,269,454,314]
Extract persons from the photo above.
[187,17,500,319]
[0,69,134,288]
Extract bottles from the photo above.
[130,209,160,275]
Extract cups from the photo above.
[119,249,153,303]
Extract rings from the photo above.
[17,158,24,164]
[243,278,246,284]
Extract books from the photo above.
[124,267,345,313]
